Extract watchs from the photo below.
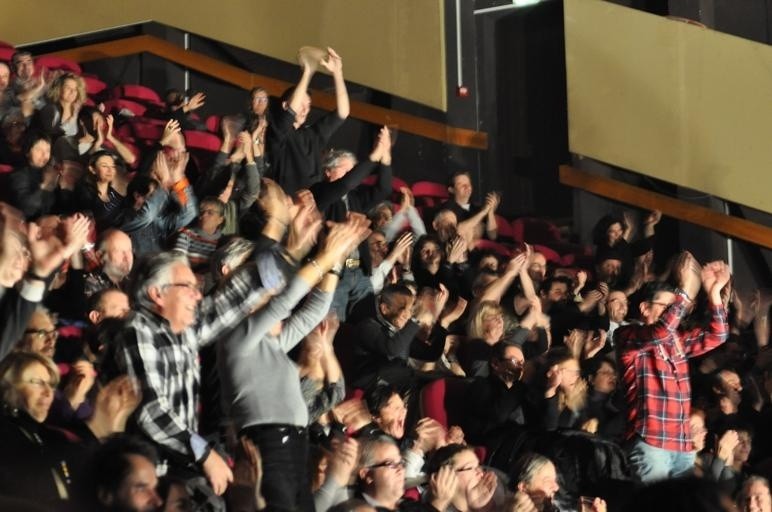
[253,137,259,147]
[330,269,343,279]
[253,137,260,146]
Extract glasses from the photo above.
[252,97,269,104]
[481,314,503,322]
[366,459,407,469]
[453,466,482,472]
[161,281,199,289]
[18,378,55,390]
[648,301,671,307]
[25,328,58,339]
[199,208,222,214]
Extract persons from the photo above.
[458,341,562,462]
[204,118,260,243]
[13,52,58,109]
[427,174,500,241]
[413,236,475,299]
[91,434,164,512]
[242,89,273,137]
[310,127,394,321]
[114,203,320,497]
[141,120,204,195]
[1,352,142,511]
[213,178,374,512]
[297,314,345,425]
[308,439,360,512]
[328,436,458,512]
[0,134,81,221]
[1,203,135,344]
[708,367,772,467]
[15,308,97,431]
[737,476,771,512]
[692,283,772,384]
[434,211,478,286]
[444,296,546,415]
[469,243,623,343]
[352,230,415,306]
[413,288,469,377]
[334,283,451,389]
[712,431,757,511]
[584,358,628,442]
[68,151,154,230]
[0,61,34,145]
[366,186,427,244]
[56,107,139,170]
[263,48,350,200]
[690,409,739,495]
[152,92,209,123]
[535,348,596,440]
[613,250,732,483]
[363,387,463,473]
[589,209,662,285]
[30,73,104,144]
[429,442,538,511]
[112,152,199,270]
[507,452,608,511]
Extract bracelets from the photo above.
[311,258,326,283]
